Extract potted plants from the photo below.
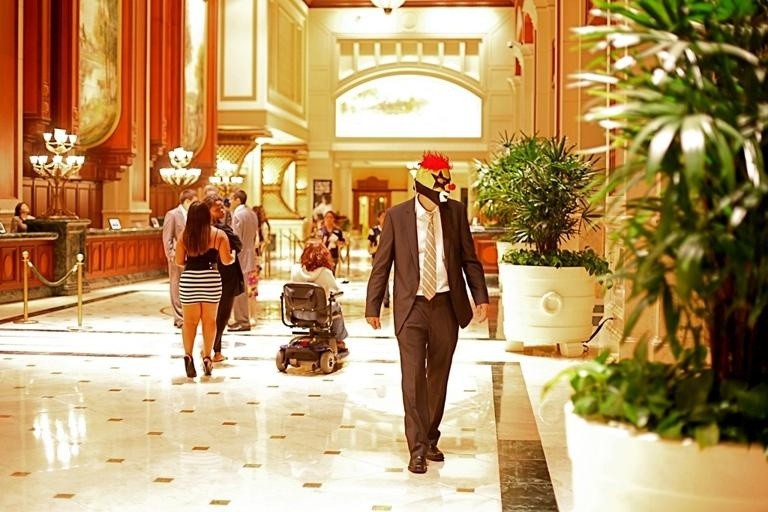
[560,0,767,511]
[470,139,538,291]
[468,126,613,349]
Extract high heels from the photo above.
[202,357,212,376]
[184,353,197,378]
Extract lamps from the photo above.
[367,0,406,13]
[209,160,244,207]
[28,129,85,220]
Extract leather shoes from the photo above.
[407,456,427,473]
[227,321,251,331]
[174,319,184,328]
[426,448,445,461]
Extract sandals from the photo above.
[212,354,229,362]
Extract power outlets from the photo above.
[159,148,202,202]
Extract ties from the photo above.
[423,211,437,302]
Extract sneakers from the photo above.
[336,342,345,349]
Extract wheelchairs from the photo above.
[275,280,349,373]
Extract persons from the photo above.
[162,184,271,377]
[317,211,345,278]
[368,210,386,267]
[312,193,332,221]
[365,150,489,473]
[11,202,36,233]
[291,243,349,350]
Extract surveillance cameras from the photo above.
[508,42,513,48]
